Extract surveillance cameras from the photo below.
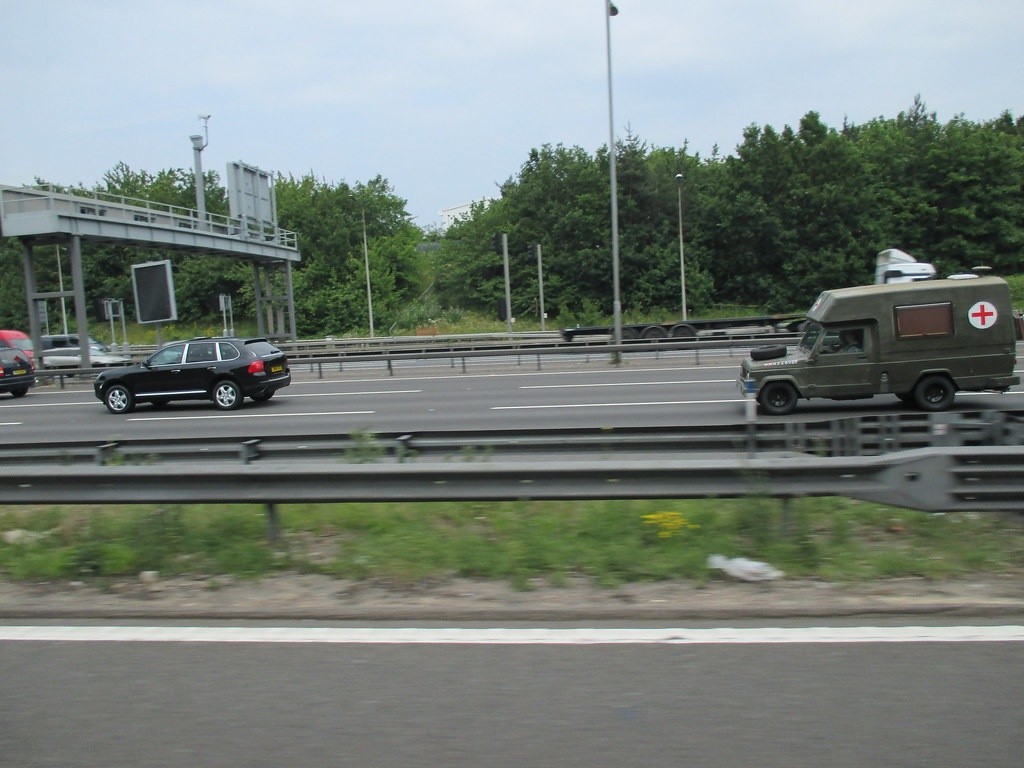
[200,114,210,119]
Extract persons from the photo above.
[830,330,862,354]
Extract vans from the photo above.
[0,329,37,376]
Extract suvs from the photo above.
[94,337,292,415]
[41,332,133,379]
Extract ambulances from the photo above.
[737,263,1021,416]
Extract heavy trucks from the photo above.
[561,248,980,352]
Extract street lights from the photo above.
[348,194,374,337]
[675,172,688,324]
[604,0,624,363]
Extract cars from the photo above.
[0,346,38,399]
